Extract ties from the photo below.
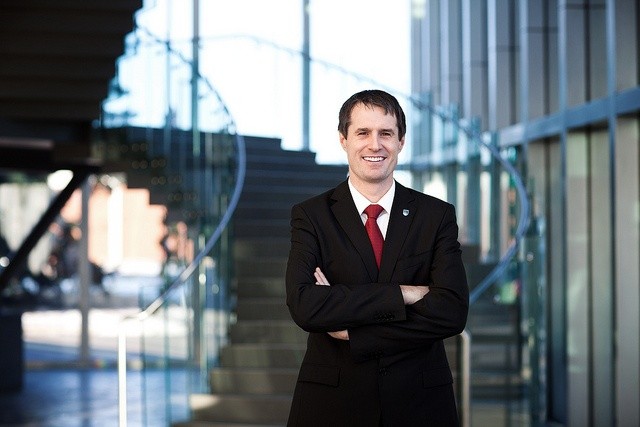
[363,204,385,272]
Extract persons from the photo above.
[285,90,470,426]
